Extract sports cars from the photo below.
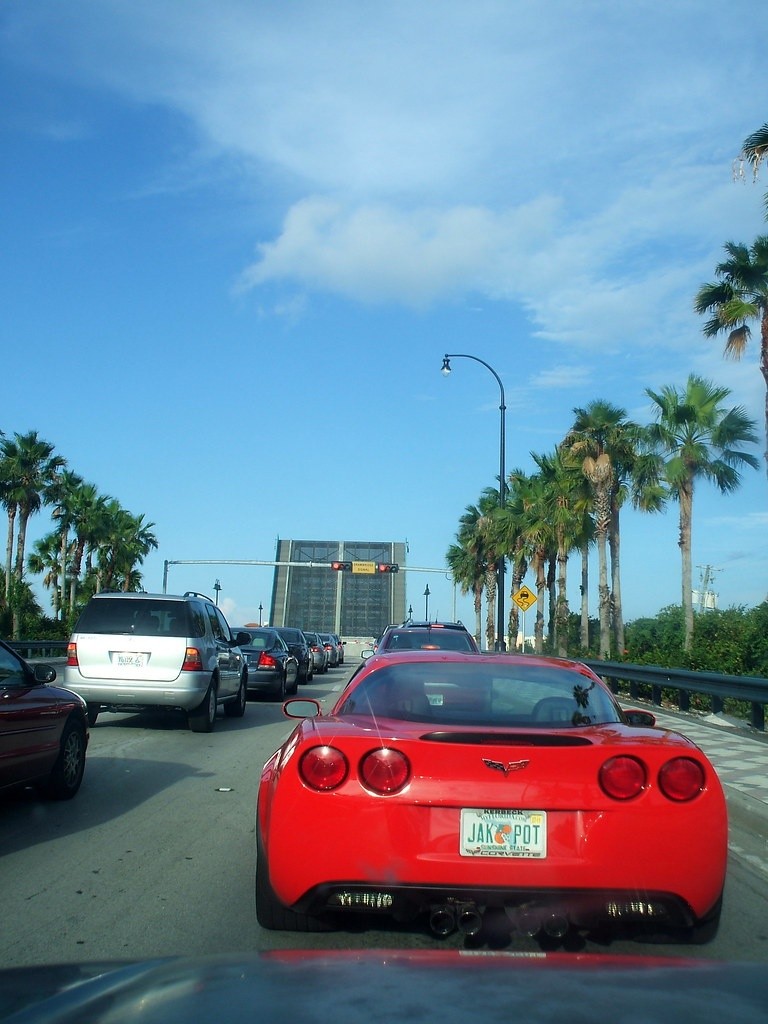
[252,649,730,941]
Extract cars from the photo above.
[0,639,91,798]
[361,619,490,711]
[304,631,331,674]
[230,628,301,702]
[317,633,342,668]
[332,634,347,664]
[267,627,317,685]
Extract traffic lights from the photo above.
[377,562,399,573]
[330,560,351,572]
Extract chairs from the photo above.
[394,637,412,649]
[530,695,595,725]
[134,610,157,633]
[252,637,266,647]
[388,689,431,715]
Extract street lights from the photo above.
[423,583,431,621]
[213,579,222,607]
[407,605,412,619]
[258,602,263,627]
[438,352,509,653]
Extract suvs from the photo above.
[64,589,253,734]
